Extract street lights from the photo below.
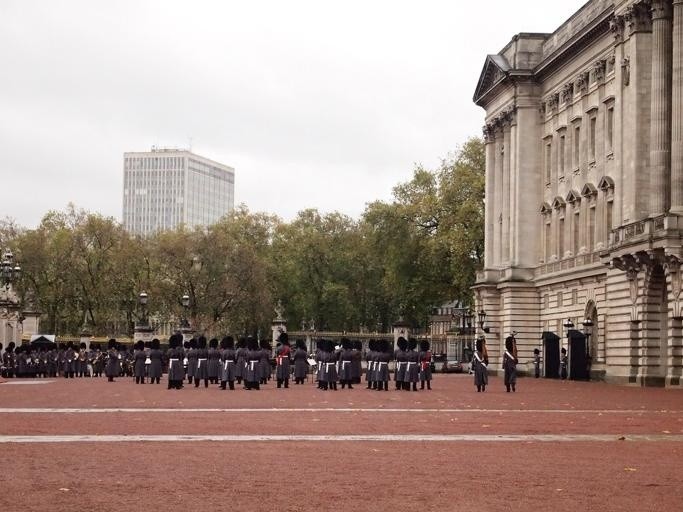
[0,248,22,292]
[565,319,574,378]
[179,295,190,328]
[581,318,593,379]
[138,290,149,326]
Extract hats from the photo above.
[399,339,408,351]
[316,336,362,351]
[407,337,417,351]
[276,334,291,347]
[507,338,516,353]
[420,339,430,352]
[168,334,272,351]
[369,338,394,352]
[397,337,404,347]
[295,339,307,352]
[6,333,160,351]
[476,337,485,351]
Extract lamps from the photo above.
[477,309,489,333]
[465,310,474,334]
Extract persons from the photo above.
[0,333,436,392]
[532,348,540,378]
[502,336,518,392]
[471,335,489,392]
[560,348,569,379]
